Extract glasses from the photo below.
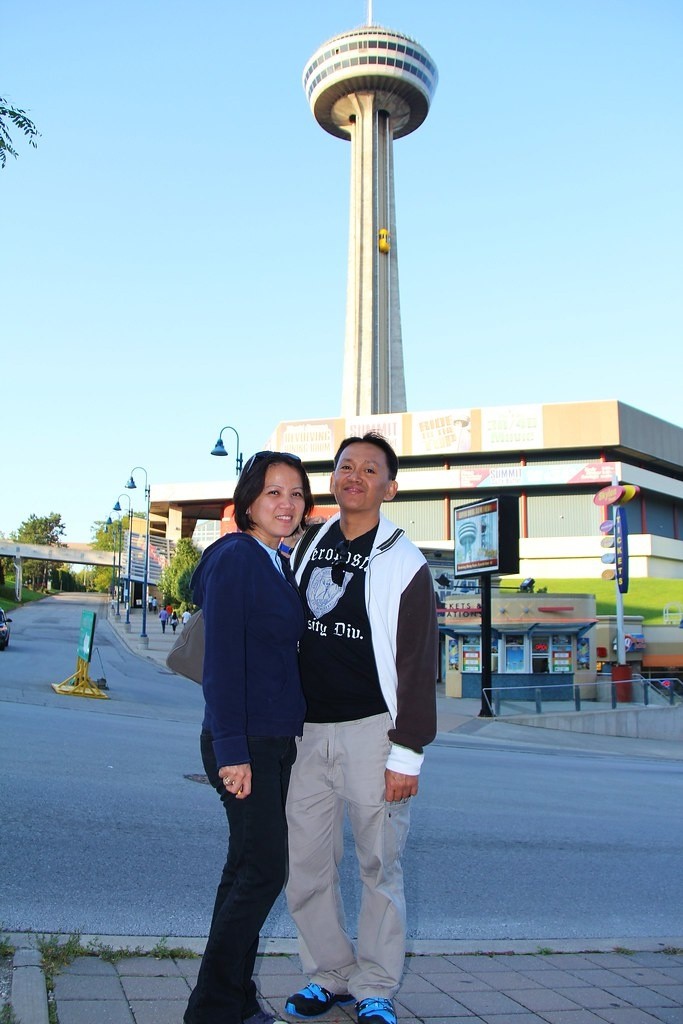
[247,451,302,474]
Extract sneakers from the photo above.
[355,997,397,1024]
[244,1010,290,1024]
[284,983,356,1018]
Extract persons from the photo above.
[170,611,179,633]
[167,603,173,624]
[182,608,191,626]
[159,607,168,633]
[148,593,153,612]
[183,450,316,1024]
[152,597,157,612]
[285,432,438,1023]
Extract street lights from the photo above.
[106,511,120,616]
[210,426,243,478]
[113,494,132,624]
[103,525,116,610]
[125,467,149,637]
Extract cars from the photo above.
[0,607,12,651]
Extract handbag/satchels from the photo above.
[166,609,205,684]
